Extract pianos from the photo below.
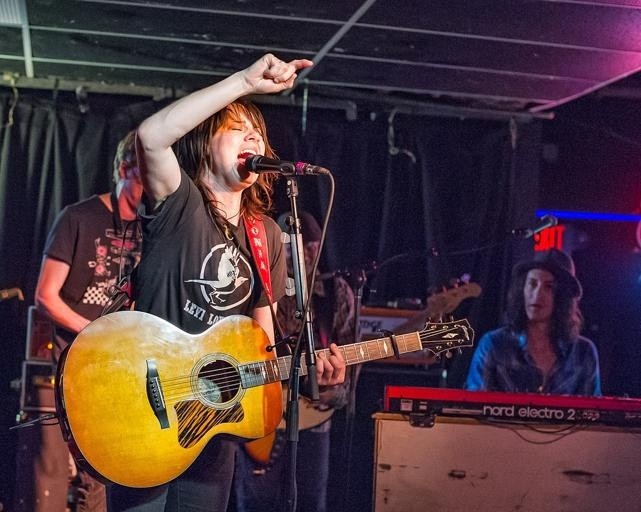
[379,383,641,430]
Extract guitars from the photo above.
[55,310,475,490]
[239,274,483,466]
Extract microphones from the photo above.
[244,155,317,175]
[523,214,559,240]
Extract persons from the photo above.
[465,248,602,396]
[105,53,346,512]
[234,210,363,512]
[33,130,143,512]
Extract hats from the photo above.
[277,211,322,242]
[512,250,583,297]
